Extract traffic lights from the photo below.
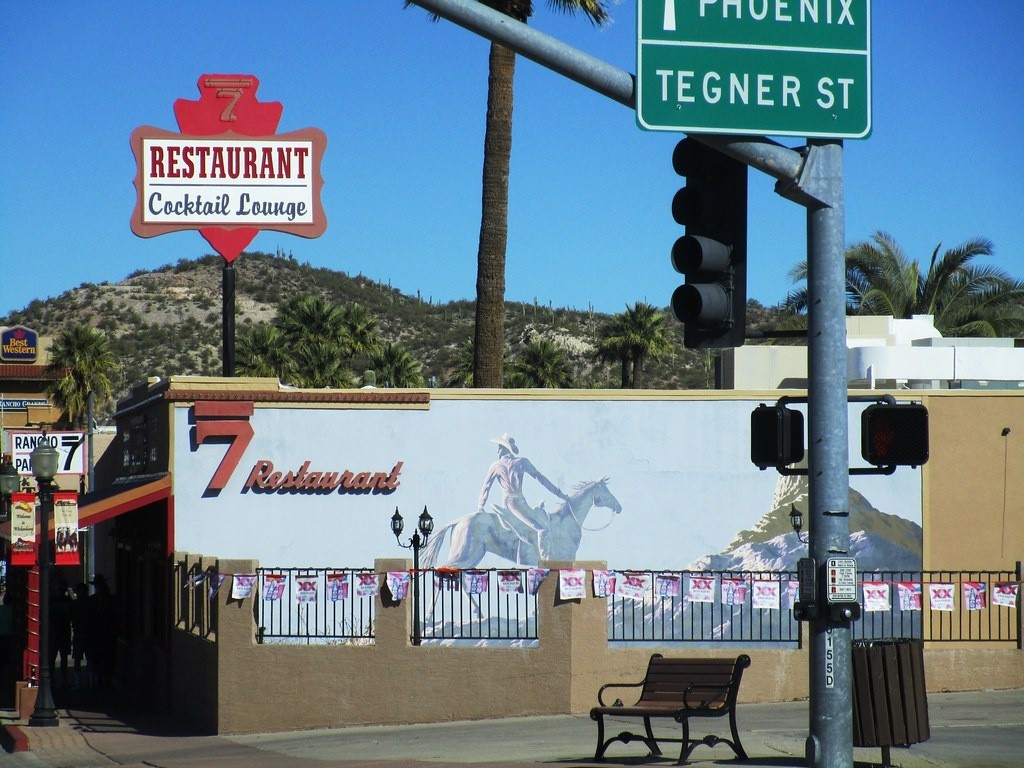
[858,403,931,468]
[749,404,805,472]
[668,135,750,349]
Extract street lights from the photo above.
[389,505,434,647]
[0,456,21,709]
[27,430,61,728]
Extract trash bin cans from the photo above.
[851,635,932,748]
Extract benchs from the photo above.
[590,653,751,766]
